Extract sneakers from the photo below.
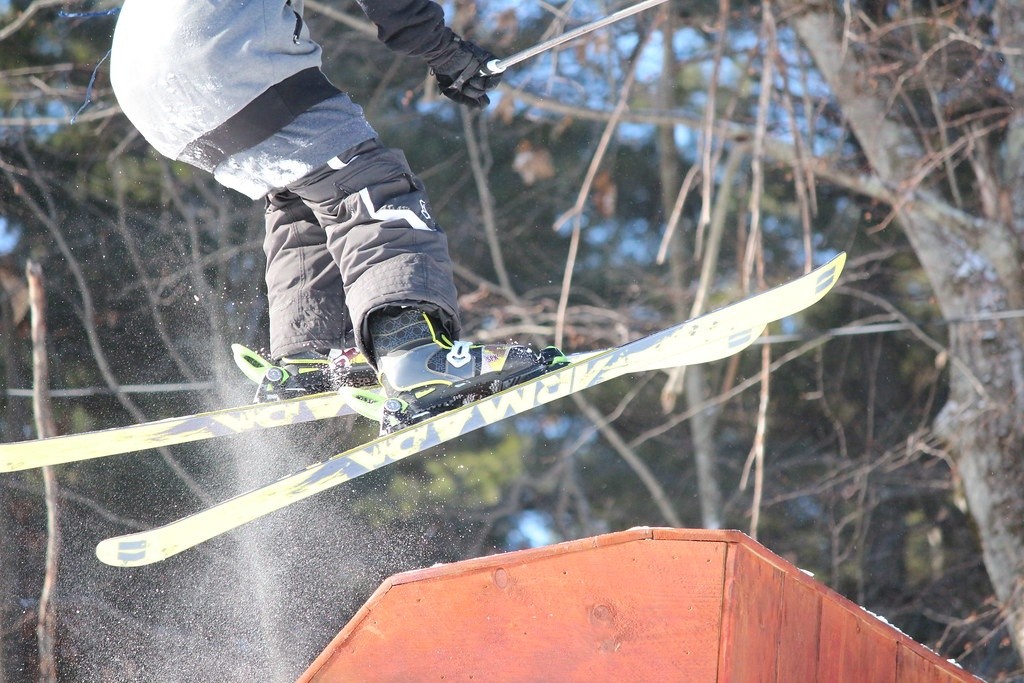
[366,308,549,436]
[253,348,380,402]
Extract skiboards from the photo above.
[0,249,850,570]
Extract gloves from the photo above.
[434,39,501,109]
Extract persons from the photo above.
[107,0,569,435]
